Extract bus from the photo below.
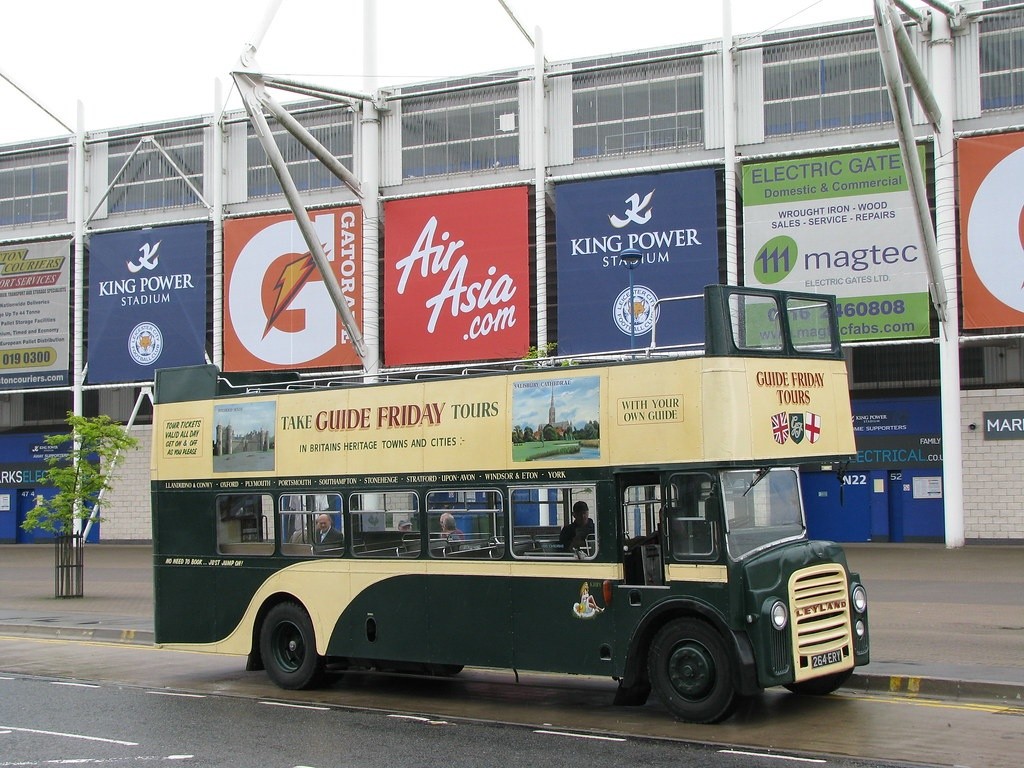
[149,286,870,726]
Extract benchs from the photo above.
[314,531,595,558]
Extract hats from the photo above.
[573,501,589,514]
[398,520,414,528]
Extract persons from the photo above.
[440,513,464,545]
[560,501,594,553]
[289,515,343,544]
[642,505,687,553]
[398,520,411,531]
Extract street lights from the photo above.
[620,247,642,354]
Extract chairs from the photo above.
[658,507,696,553]
